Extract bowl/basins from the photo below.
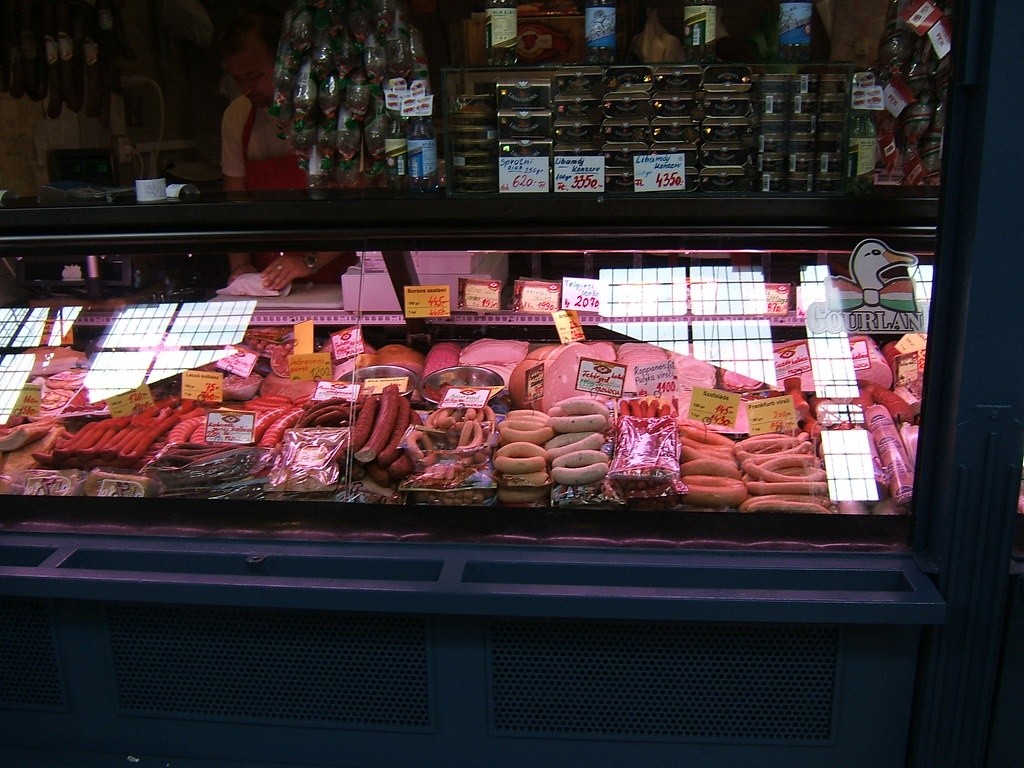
[418,366,503,409]
[338,365,417,401]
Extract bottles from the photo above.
[167,183,198,199]
[405,118,439,194]
[844,109,877,192]
[381,121,406,194]
[777,1,813,63]
[683,1,718,63]
[485,0,520,67]
[585,2,616,64]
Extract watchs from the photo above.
[304,252,321,275]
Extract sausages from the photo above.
[30,396,914,515]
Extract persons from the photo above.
[213,15,364,290]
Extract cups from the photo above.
[134,177,168,201]
